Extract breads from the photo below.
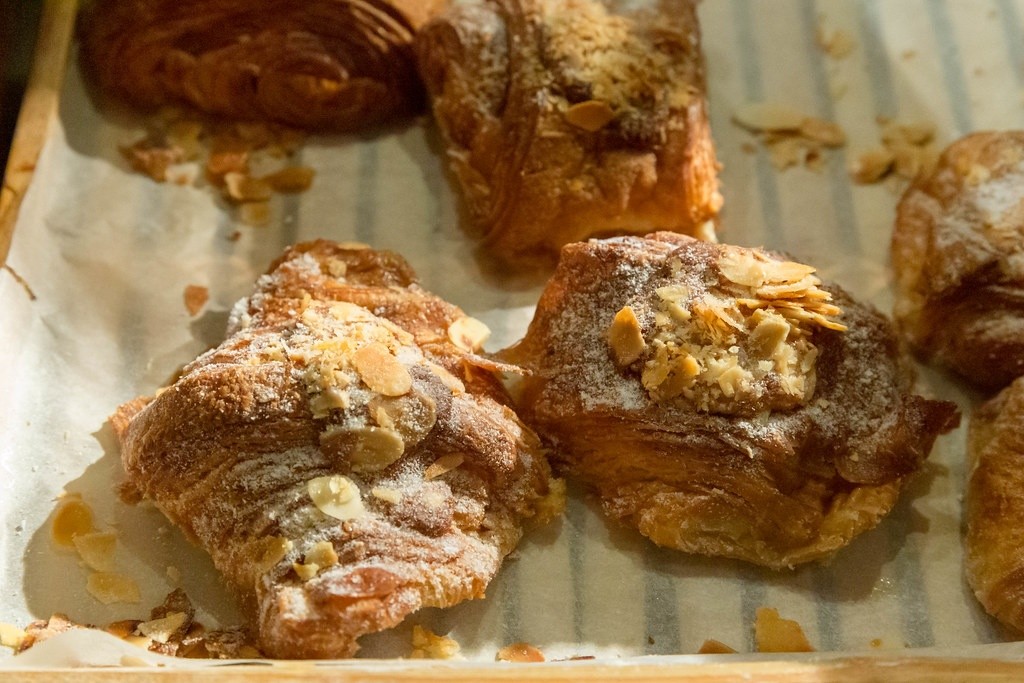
[75,0,1024,658]
